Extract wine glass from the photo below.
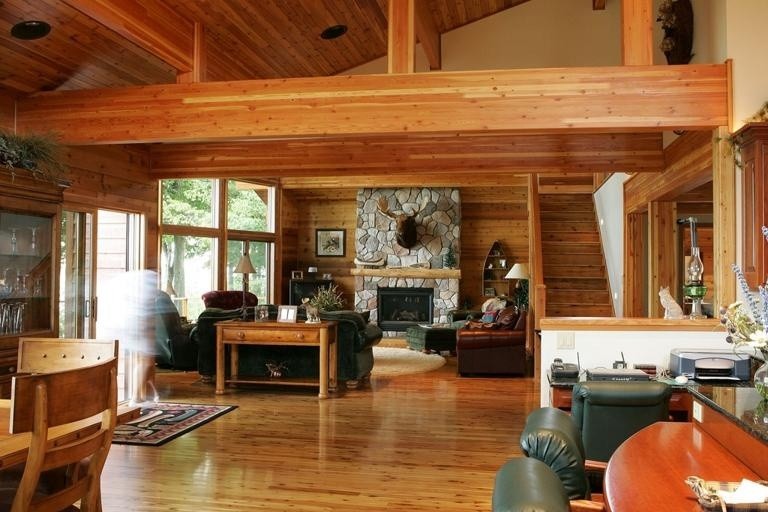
[0,302,27,334]
[7,224,20,251]
[0,265,44,299]
[27,225,40,252]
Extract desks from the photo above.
[602,384,768,512]
[215,321,340,399]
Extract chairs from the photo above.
[153,291,259,370]
[571,380,673,462]
[518,407,609,507]
[0,336,122,512]
[492,458,607,512]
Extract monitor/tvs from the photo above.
[288,280,334,317]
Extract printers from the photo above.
[668,349,750,381]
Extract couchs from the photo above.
[447,298,528,377]
[190,308,381,386]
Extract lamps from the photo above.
[233,252,257,322]
[505,263,530,305]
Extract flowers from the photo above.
[719,226,768,366]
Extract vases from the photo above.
[754,360,768,400]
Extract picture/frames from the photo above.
[315,228,346,258]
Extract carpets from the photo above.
[367,346,445,378]
[109,401,239,447]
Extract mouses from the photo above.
[674,376,688,384]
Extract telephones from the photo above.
[551,352,580,378]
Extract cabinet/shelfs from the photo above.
[289,279,335,310]
[1,184,61,399]
[481,239,510,301]
